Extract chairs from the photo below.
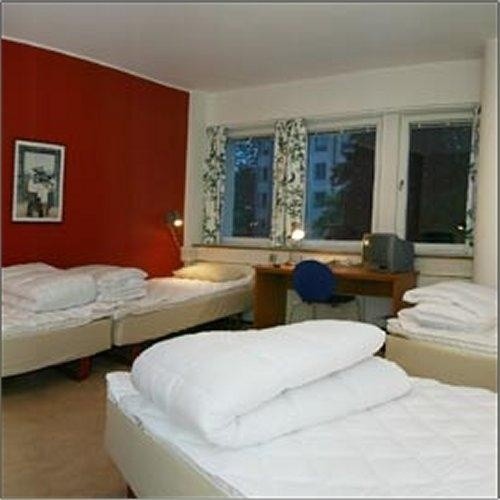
[289,260,361,324]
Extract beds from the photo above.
[1,261,254,381]
[381,279,498,389]
[104,321,499,499]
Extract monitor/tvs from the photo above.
[361,232,413,275]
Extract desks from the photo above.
[254,263,415,324]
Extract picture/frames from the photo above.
[11,137,64,225]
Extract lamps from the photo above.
[165,212,186,247]
[285,223,307,242]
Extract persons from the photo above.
[24,165,55,217]
